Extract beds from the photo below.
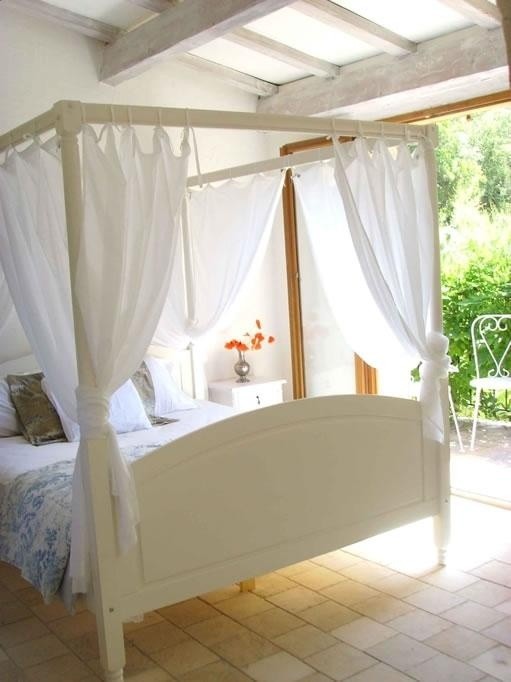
[1,100,453,680]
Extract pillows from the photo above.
[1,354,199,438]
[40,367,153,443]
[6,360,180,446]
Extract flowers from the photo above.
[224,320,274,351]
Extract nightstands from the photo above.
[208,378,288,411]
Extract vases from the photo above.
[234,351,251,384]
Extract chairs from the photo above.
[470,314,511,450]
